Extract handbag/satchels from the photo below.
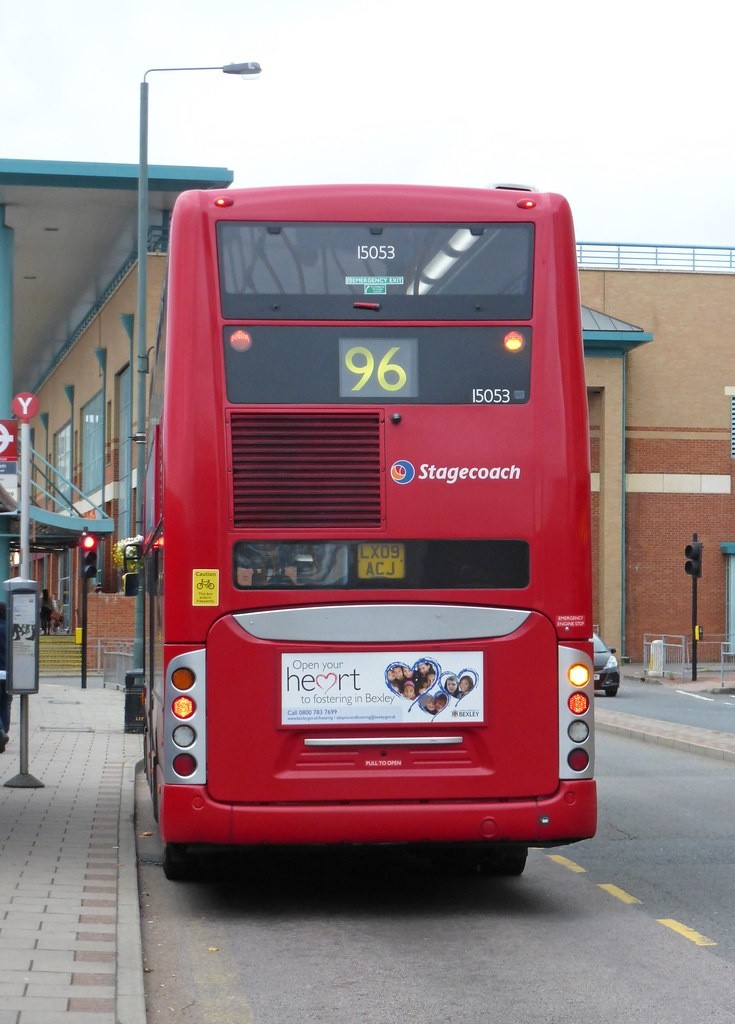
[50,611,60,621]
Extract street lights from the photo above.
[135,62,263,686]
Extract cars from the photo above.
[593,632,621,697]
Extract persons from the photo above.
[0,604,13,753]
[387,663,473,715]
[38,589,62,636]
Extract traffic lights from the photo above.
[695,625,703,640]
[683,543,703,577]
[81,536,98,577]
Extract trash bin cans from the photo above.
[648,640,665,676]
[123,669,146,733]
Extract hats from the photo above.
[404,681,414,686]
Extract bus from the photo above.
[125,183,597,880]
